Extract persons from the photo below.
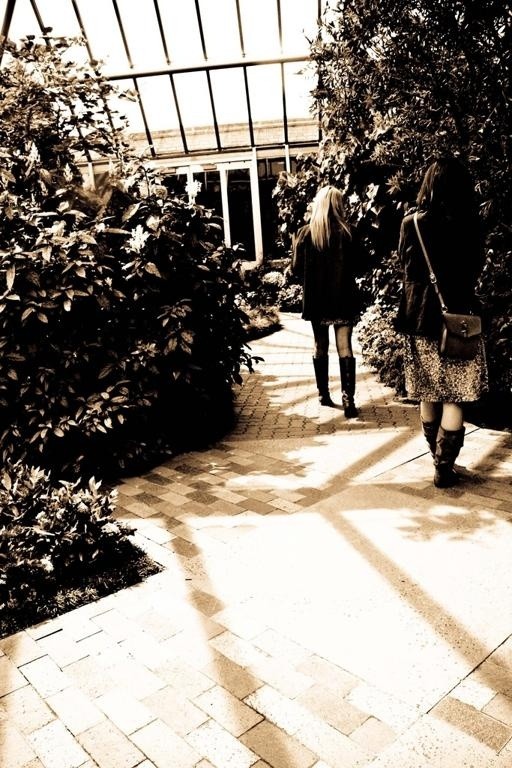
[290,184,373,418]
[396,158,486,487]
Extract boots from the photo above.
[339,356,358,417]
[420,415,465,488]
[313,355,333,406]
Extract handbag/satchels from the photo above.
[441,313,483,361]
